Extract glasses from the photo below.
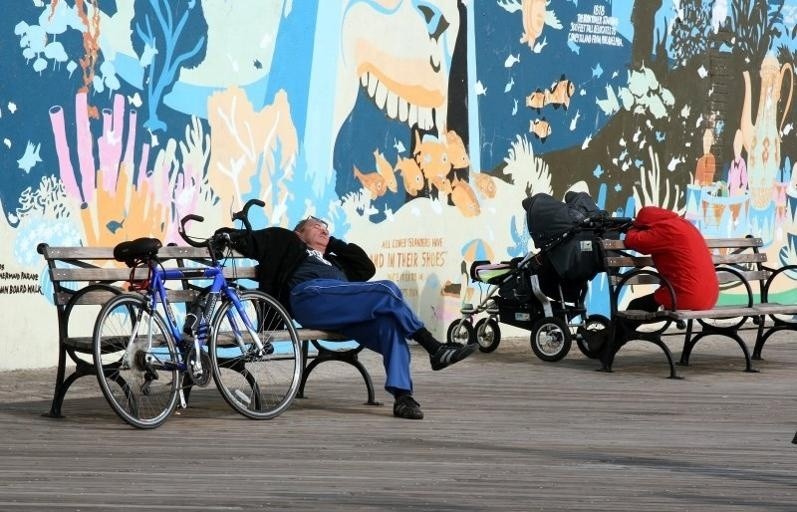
[304,216,328,228]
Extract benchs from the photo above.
[35,238,386,417]
[594,231,797,380]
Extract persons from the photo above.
[577,206,719,361]
[213,215,480,419]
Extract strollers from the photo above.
[443,190,633,363]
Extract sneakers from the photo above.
[580,328,603,350]
[430,342,480,370]
[394,396,423,419]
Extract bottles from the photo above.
[183,297,205,334]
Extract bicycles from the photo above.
[95,194,308,430]
